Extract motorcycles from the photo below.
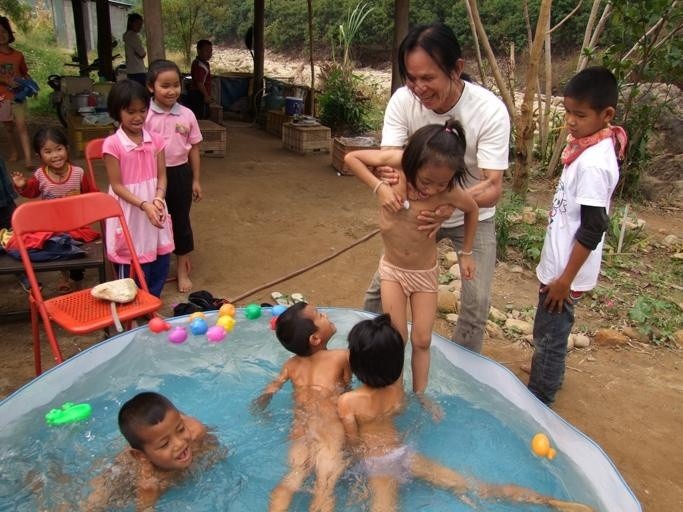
[45,39,127,128]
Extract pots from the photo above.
[70,92,100,111]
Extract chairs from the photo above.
[207,78,223,122]
[10,188,163,379]
[84,137,181,287]
[55,76,118,153]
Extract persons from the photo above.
[29,393,208,512]
[364,23,510,351]
[0,158,43,292]
[124,10,147,85]
[146,60,202,292]
[526,66,628,406]
[345,119,478,426]
[337,313,595,512]
[11,126,89,294]
[0,15,40,169]
[250,301,351,512]
[101,79,176,326]
[190,40,215,120]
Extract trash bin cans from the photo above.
[211,76,254,114]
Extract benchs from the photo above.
[0,229,114,345]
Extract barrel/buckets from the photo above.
[90,82,115,108]
[285,97,304,116]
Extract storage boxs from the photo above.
[331,136,381,176]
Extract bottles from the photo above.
[293,103,299,122]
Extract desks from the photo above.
[265,111,312,137]
[196,120,229,158]
[280,122,332,156]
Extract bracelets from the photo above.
[373,180,383,196]
[154,198,167,208]
[458,250,474,256]
[139,201,148,212]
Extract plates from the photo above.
[303,121,315,124]
